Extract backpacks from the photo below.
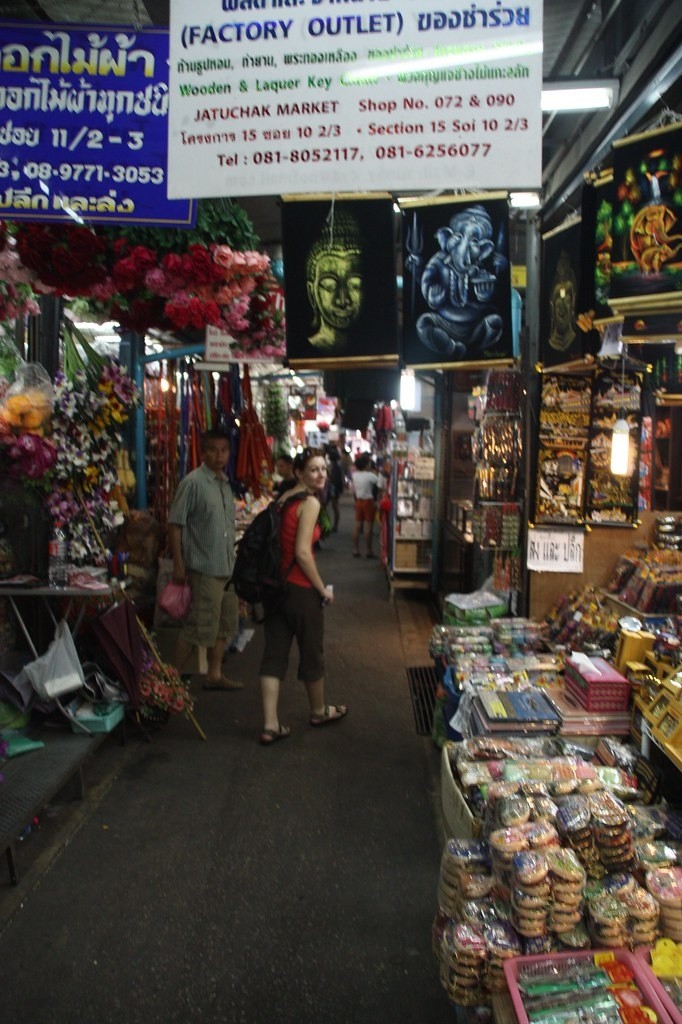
[224,489,322,604]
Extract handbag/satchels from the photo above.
[25,618,84,702]
[161,574,192,622]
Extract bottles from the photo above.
[49,522,68,589]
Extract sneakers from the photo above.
[208,676,245,691]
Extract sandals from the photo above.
[261,725,290,745]
[310,704,348,725]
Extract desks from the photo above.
[0,585,113,735]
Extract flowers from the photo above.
[0,198,288,727]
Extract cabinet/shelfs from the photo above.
[380,44,682,1024]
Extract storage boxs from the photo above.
[442,594,510,627]
[634,945,682,1024]
[71,704,125,733]
[439,737,622,841]
[395,541,418,569]
[502,948,674,1024]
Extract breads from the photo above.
[432,764,682,1006]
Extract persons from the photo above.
[166,430,245,691]
[267,440,379,559]
[256,446,349,745]
